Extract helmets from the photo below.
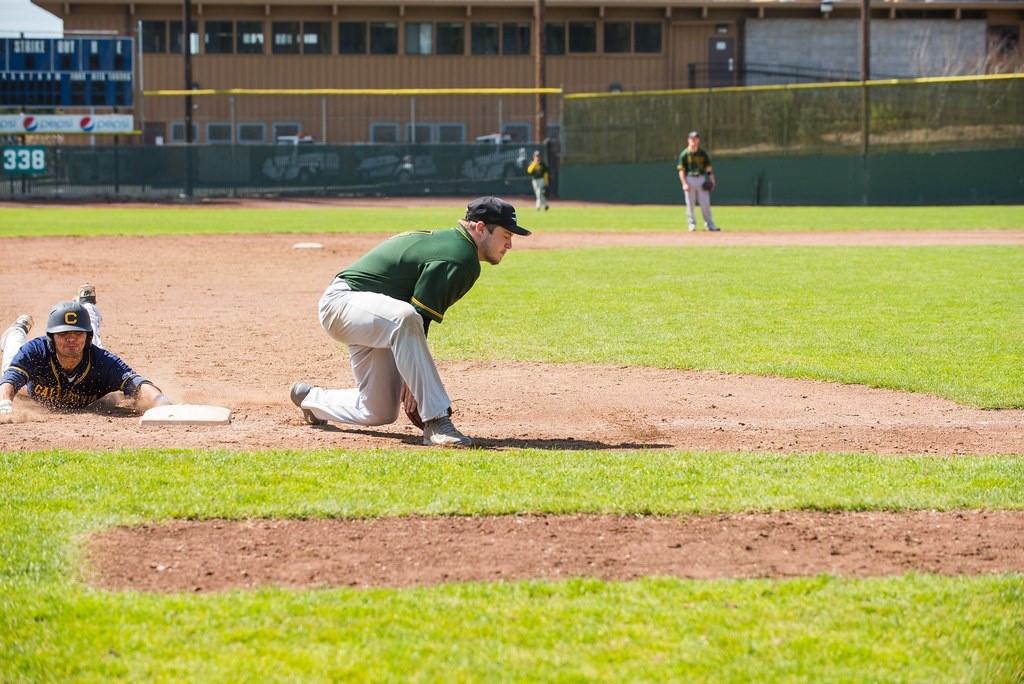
[48,302,93,333]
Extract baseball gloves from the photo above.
[702,181,714,190]
[405,392,453,430]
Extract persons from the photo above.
[527,151,552,211]
[676,131,721,233]
[1,282,171,421]
[289,193,532,448]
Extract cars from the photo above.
[357,141,437,181]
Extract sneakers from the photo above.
[423,418,473,447]
[73,284,97,304]
[290,381,327,424]
[689,225,697,231]
[706,225,720,231]
[1,315,34,351]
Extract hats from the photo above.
[465,194,532,235]
[534,150,541,156]
[688,131,701,138]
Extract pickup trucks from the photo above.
[264,134,340,186]
[463,134,549,183]
